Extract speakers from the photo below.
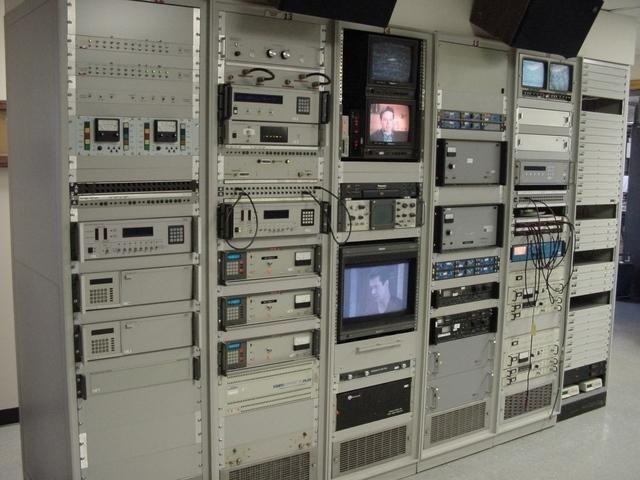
[253,0,398,28]
[469,1,605,57]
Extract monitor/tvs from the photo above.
[366,33,419,97]
[518,53,577,102]
[338,240,421,342]
[362,97,418,162]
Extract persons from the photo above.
[371,105,405,142]
[360,269,404,315]
